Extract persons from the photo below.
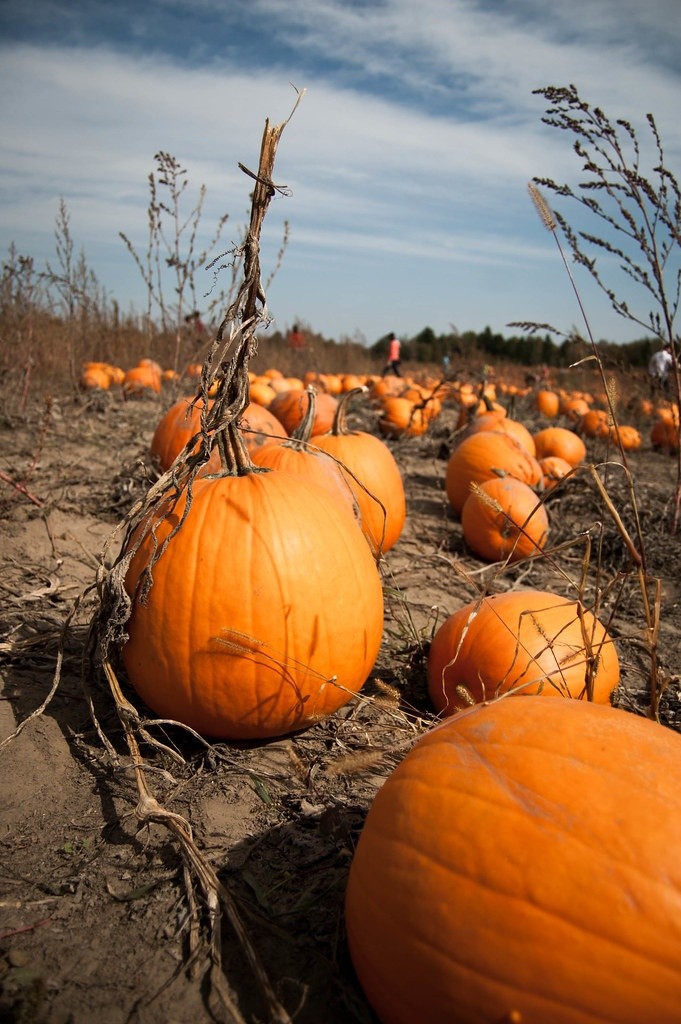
[290,325,305,346]
[649,344,681,400]
[381,333,404,378]
[191,311,207,337]
[442,353,450,375]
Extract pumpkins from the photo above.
[426,590,620,718]
[81,358,681,560]
[118,414,384,740]
[343,691,681,1024]
